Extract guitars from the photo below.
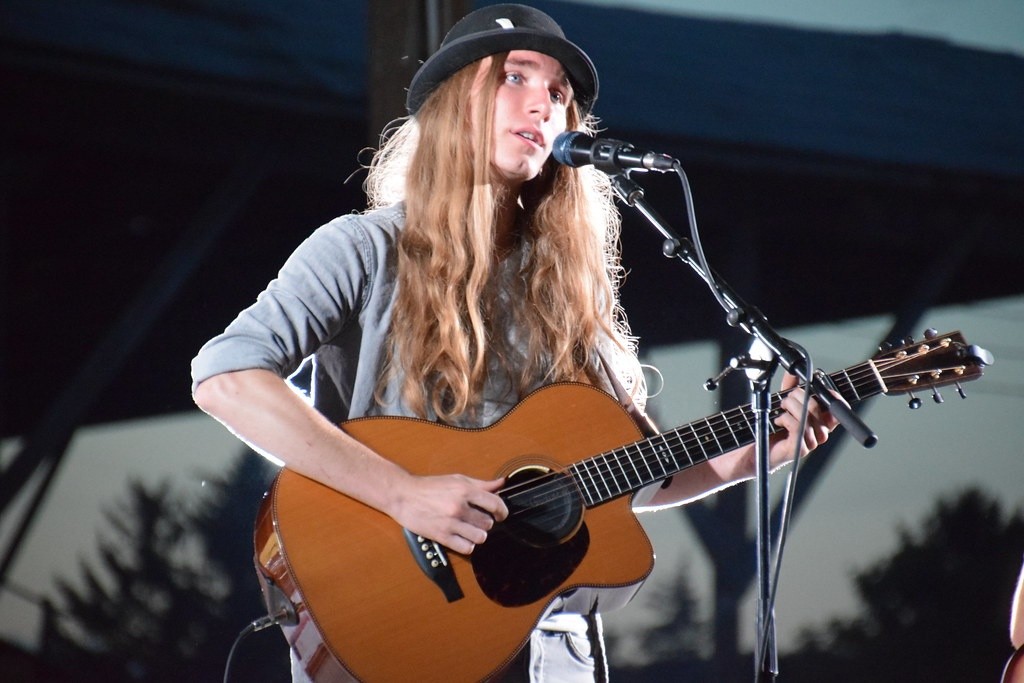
[253,324,994,682]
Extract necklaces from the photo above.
[480,295,498,331]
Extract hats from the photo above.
[408,4,597,121]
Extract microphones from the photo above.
[552,131,680,173]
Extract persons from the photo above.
[190,3,844,683]
